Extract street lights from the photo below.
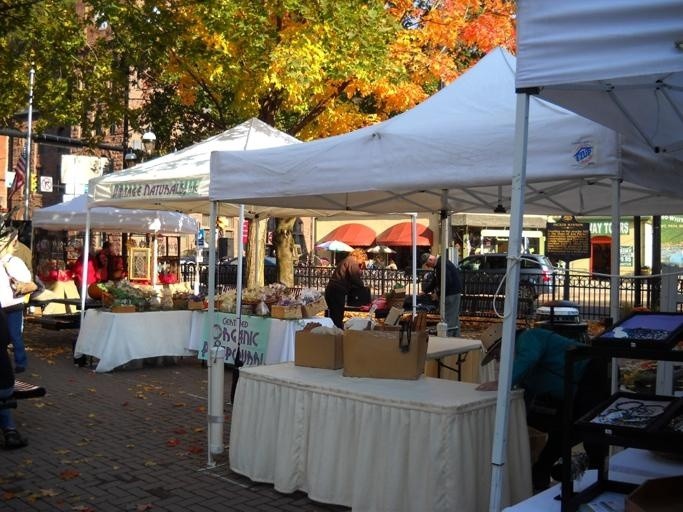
[124,128,157,169]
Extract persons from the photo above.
[420,253,462,338]
[324,247,369,331]
[0,215,124,451]
[370,256,386,290]
[384,260,397,288]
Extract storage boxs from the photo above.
[302,296,328,317]
[272,305,302,318]
[343,331,428,380]
[295,330,342,370]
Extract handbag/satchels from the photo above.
[347,286,371,306]
[10,277,38,298]
[32,275,45,298]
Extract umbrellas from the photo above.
[366,244,397,269]
[315,240,355,270]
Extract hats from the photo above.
[479,323,526,366]
[420,253,429,264]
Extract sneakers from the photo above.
[15,367,25,372]
[3,429,29,448]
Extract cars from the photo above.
[178,248,294,287]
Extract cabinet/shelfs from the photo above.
[562,346,683,512]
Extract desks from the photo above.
[229,363,533,512]
[503,468,653,512]
[188,309,334,373]
[419,336,483,381]
[73,307,199,373]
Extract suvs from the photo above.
[422,252,556,302]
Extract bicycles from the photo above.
[295,264,412,290]
